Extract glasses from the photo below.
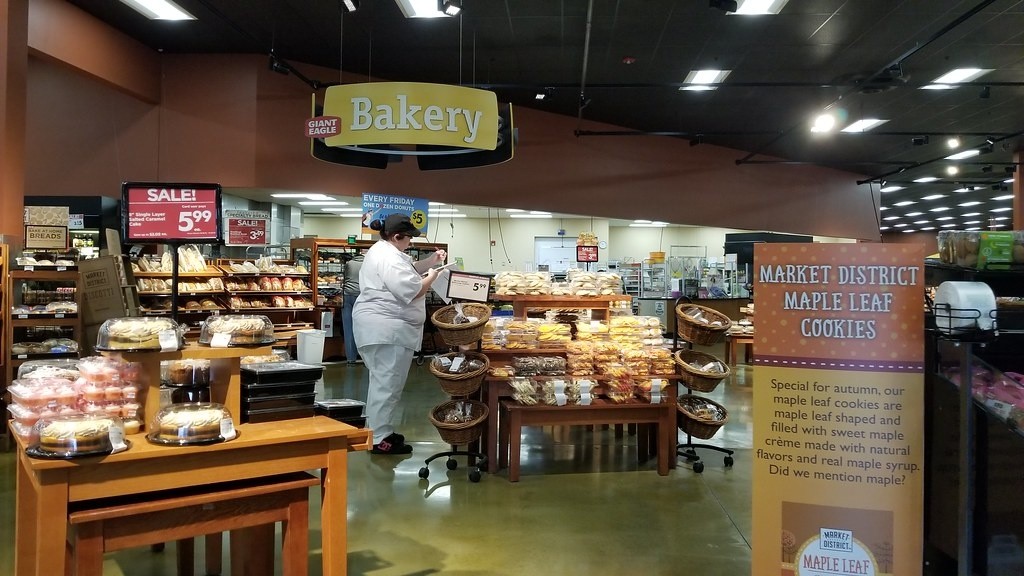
[401,234,413,240]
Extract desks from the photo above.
[724,331,754,367]
[8,414,372,576]
[501,398,674,481]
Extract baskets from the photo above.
[429,352,490,396]
[431,303,491,346]
[676,394,729,439]
[429,400,489,446]
[676,303,732,346]
[674,349,731,393]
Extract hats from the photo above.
[381,213,421,237]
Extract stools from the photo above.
[66,471,319,576]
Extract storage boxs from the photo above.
[935,229,1024,269]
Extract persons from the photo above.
[342,253,365,365]
[354,213,447,454]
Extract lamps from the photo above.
[114,0,1023,193]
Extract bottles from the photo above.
[72,234,94,247]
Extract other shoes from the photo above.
[347,361,356,366]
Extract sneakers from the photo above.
[370,432,413,454]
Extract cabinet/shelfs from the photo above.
[290,237,448,361]
[133,258,317,348]
[615,262,667,315]
[481,293,683,471]
[5,270,83,445]
